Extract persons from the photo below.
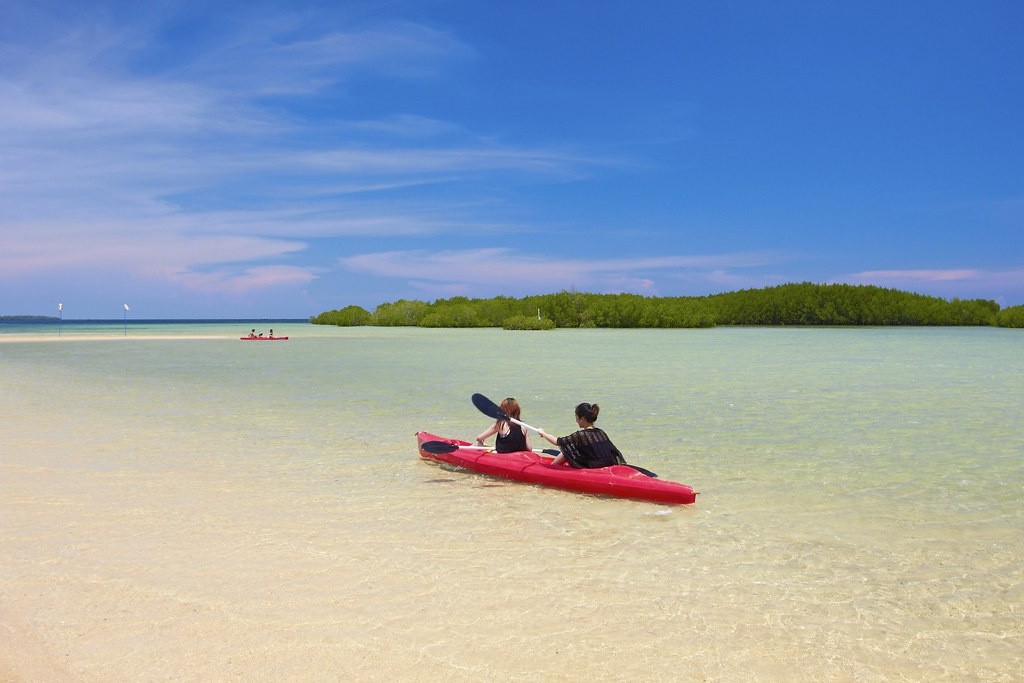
[269,329,274,338]
[538,403,627,469]
[477,398,532,453]
[248,329,257,337]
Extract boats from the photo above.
[240,336,289,341]
[415,431,700,505]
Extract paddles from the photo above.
[258,333,263,336]
[470,392,659,478]
[421,440,561,457]
